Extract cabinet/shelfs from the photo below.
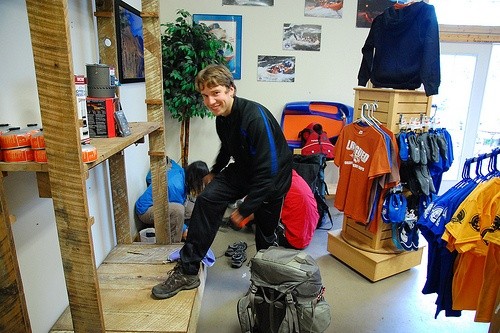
[0,0,208,333]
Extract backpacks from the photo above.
[291,152,328,227]
[236,246,332,333]
[299,122,336,159]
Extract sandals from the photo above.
[225,241,247,256]
[231,250,247,268]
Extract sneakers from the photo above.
[151,264,200,299]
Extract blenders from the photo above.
[79,99,88,136]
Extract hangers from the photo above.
[353,102,382,129]
[454,147,500,188]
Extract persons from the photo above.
[135,161,209,243]
[246,169,318,250]
[152,65,293,299]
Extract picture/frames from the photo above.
[115,0,145,84]
[193,13,242,79]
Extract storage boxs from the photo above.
[85,97,119,138]
